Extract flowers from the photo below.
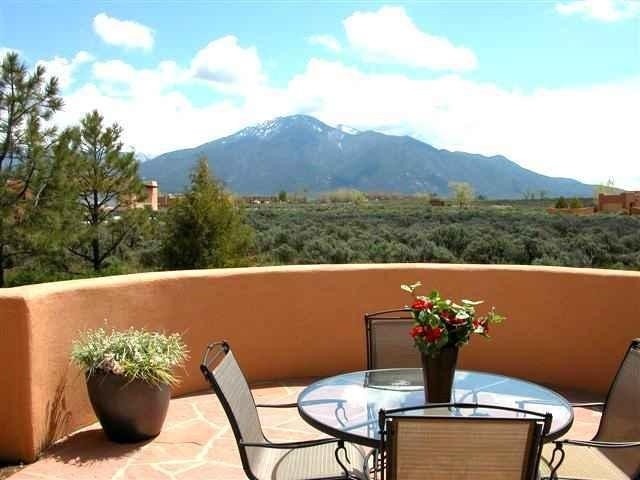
[397,281,508,360]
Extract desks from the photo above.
[294,364,576,478]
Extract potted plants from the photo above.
[67,328,193,445]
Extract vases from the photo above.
[414,347,460,402]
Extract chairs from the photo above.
[201,337,370,478]
[365,312,463,370]
[362,399,555,478]
[514,336,638,478]
